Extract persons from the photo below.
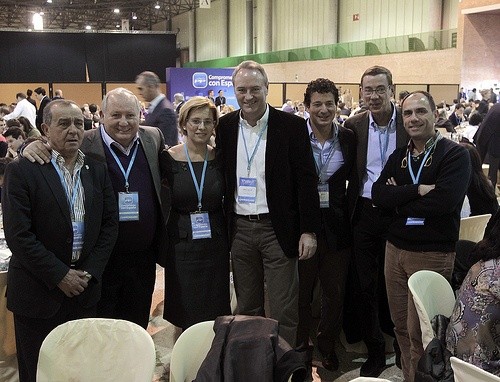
[213,60,499,382]
[0,70,235,376]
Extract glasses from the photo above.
[186,118,217,128]
[361,84,391,95]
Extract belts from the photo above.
[235,212,269,221]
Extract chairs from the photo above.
[34,317,157,382]
[170,318,295,382]
[450,356,500,382]
[409,270,456,352]
[460,213,492,245]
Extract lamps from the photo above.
[155,1,160,9]
[132,12,137,20]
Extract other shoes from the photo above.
[359,343,386,377]
[393,340,402,371]
[322,350,340,370]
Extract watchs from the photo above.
[84,271,93,280]
